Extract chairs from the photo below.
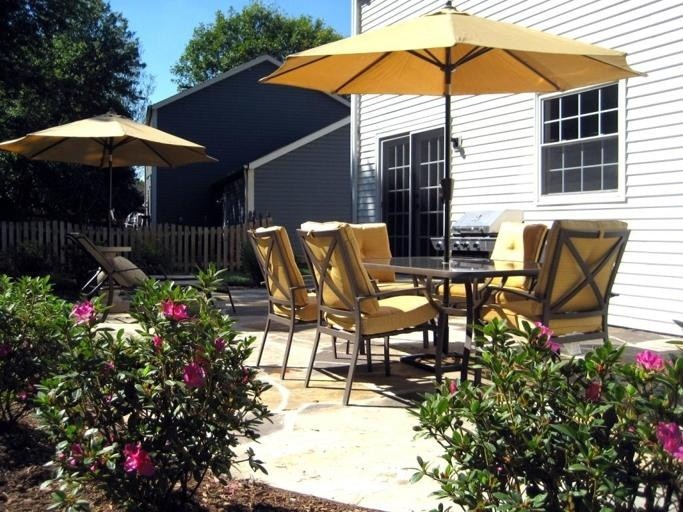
[297,222,449,405]
[247,226,339,380]
[66,233,235,321]
[474,220,631,387]
[438,222,548,361]
[346,222,435,378]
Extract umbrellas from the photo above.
[0,107,221,245]
[254,0,649,354]
